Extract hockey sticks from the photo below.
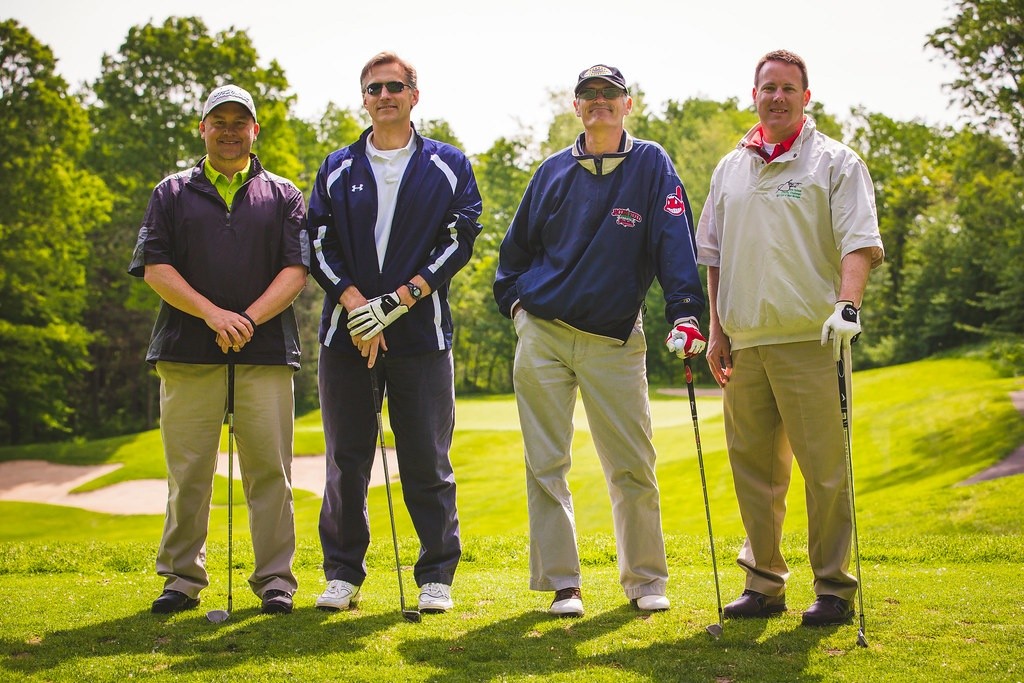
[369,364,421,623]
[206,347,235,623]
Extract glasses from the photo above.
[576,87,624,100]
[368,82,412,95]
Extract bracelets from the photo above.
[239,309,257,330]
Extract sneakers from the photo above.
[417,582,452,610]
[316,579,362,608]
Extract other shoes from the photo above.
[549,588,583,616]
[636,594,671,610]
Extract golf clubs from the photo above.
[674,337,724,638]
[828,330,869,647]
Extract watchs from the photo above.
[403,281,423,303]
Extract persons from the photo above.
[124,86,312,612]
[493,65,707,618]
[310,50,486,613]
[696,51,886,624]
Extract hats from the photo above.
[203,85,257,123]
[575,65,628,97]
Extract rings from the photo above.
[232,344,239,348]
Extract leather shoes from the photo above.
[261,589,293,612]
[151,590,200,611]
[724,590,788,617]
[802,595,856,626]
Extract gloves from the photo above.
[823,302,863,361]
[345,290,409,341]
[665,322,706,359]
